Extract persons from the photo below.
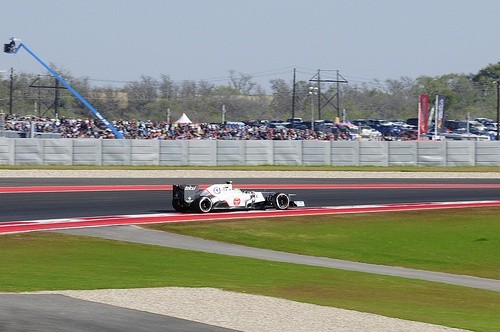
[0,111,499,144]
[226,180,241,191]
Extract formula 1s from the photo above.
[227,110,499,143]
[174,180,299,213]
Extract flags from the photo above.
[420,95,429,134]
[437,95,446,134]
